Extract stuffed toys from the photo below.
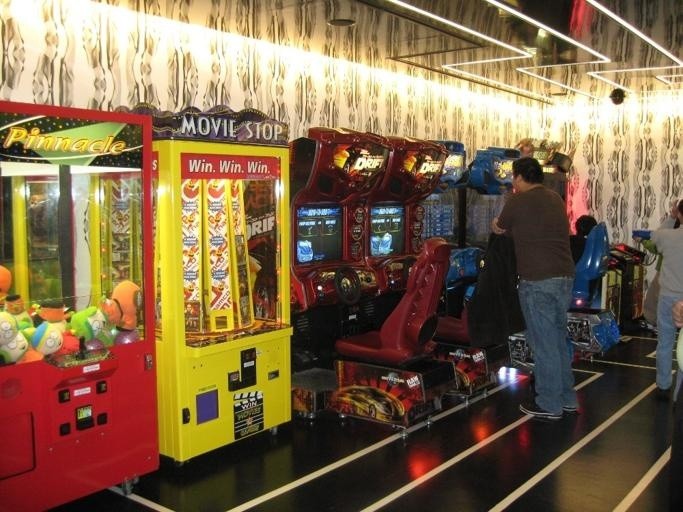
[1,264,142,364]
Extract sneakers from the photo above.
[560,404,578,412]
[519,400,562,419]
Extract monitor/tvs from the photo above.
[465,193,509,246]
[369,205,406,257]
[419,188,459,241]
[296,206,344,264]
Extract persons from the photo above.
[492,157,580,421]
[631,233,663,326]
[670,299,683,512]
[650,198,683,401]
[570,213,597,265]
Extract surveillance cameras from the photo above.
[610,96,624,105]
[611,88,624,96]
